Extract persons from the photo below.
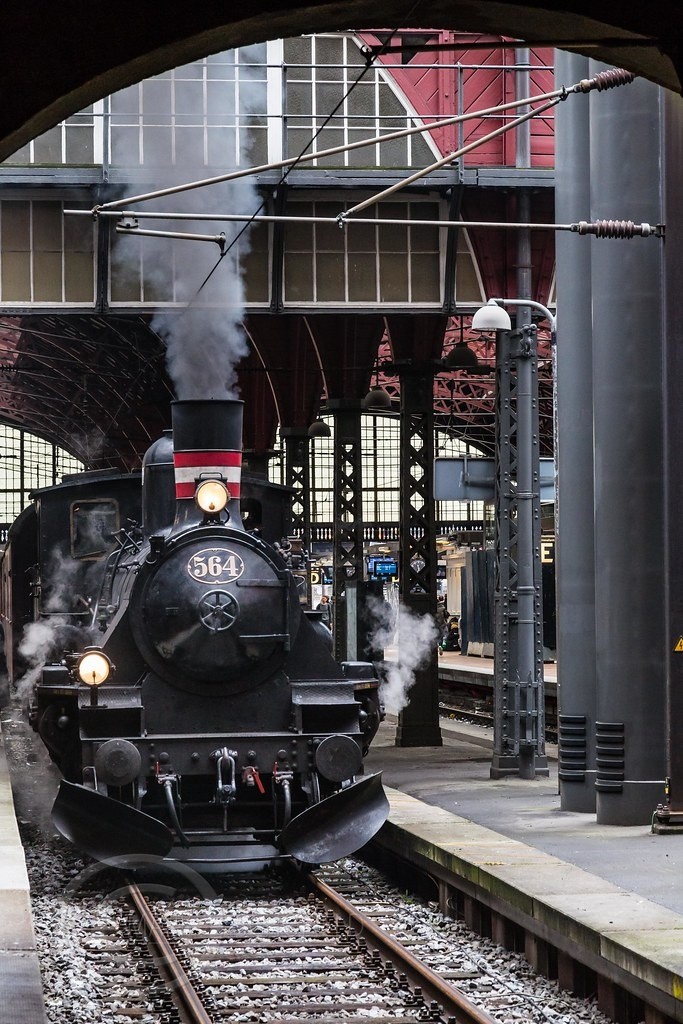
[316,596,333,632]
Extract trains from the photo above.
[0,397,390,876]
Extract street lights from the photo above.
[471,298,560,563]
